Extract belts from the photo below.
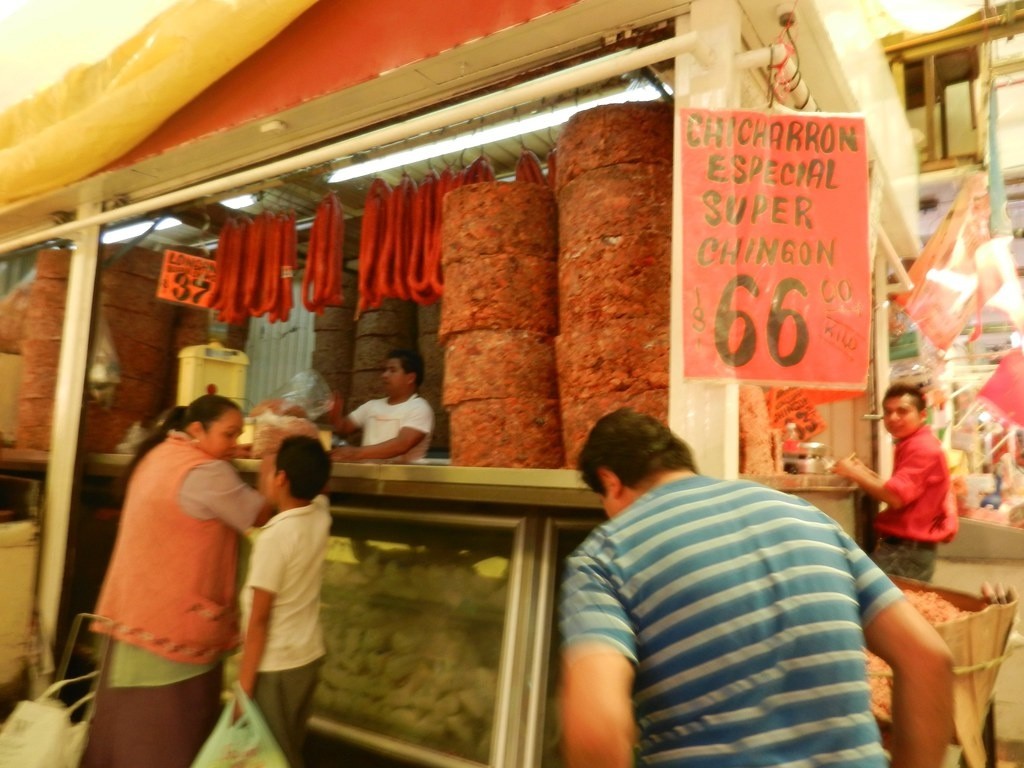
[887,537,936,551]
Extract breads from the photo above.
[309,551,508,761]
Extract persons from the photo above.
[557,407,955,768]
[325,347,435,462]
[233,433,334,768]
[75,394,274,768]
[829,381,959,581]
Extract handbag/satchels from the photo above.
[187,684,291,768]
[0,670,103,768]
